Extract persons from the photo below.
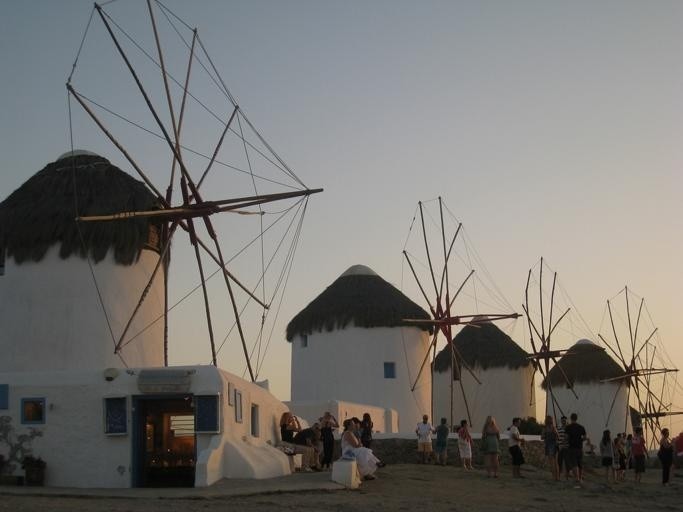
[416,415,436,464]
[657,428,674,485]
[279,411,384,481]
[599,428,649,485]
[507,417,527,478]
[458,420,473,470]
[435,416,449,465]
[542,413,587,490]
[482,415,502,479]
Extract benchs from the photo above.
[331,456,358,487]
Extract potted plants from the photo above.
[22,455,47,487]
[0,416,42,485]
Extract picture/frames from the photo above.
[193,392,220,434]
[235,389,243,423]
[102,394,128,436]
